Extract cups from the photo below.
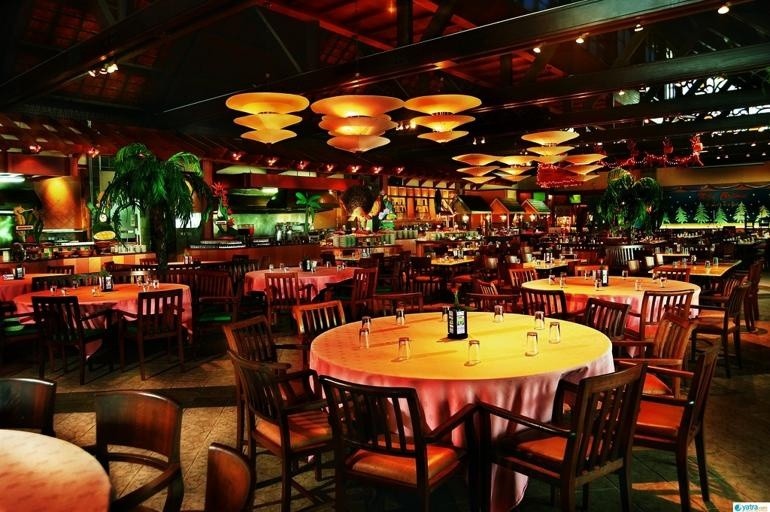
[515,256,566,266]
[269,261,347,273]
[671,232,698,239]
[744,237,755,244]
[49,276,160,297]
[111,245,146,253]
[672,255,719,268]
[549,269,667,291]
[655,246,689,254]
[430,250,467,263]
[359,305,561,365]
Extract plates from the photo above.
[3,274,16,279]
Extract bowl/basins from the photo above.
[75,246,93,257]
[37,241,53,260]
[53,246,76,257]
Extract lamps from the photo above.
[493,143,540,183]
[564,143,608,182]
[451,152,501,184]
[310,72,405,156]
[575,33,590,44]
[402,78,483,144]
[533,42,544,54]
[225,73,309,148]
[634,23,644,32]
[521,116,580,164]
